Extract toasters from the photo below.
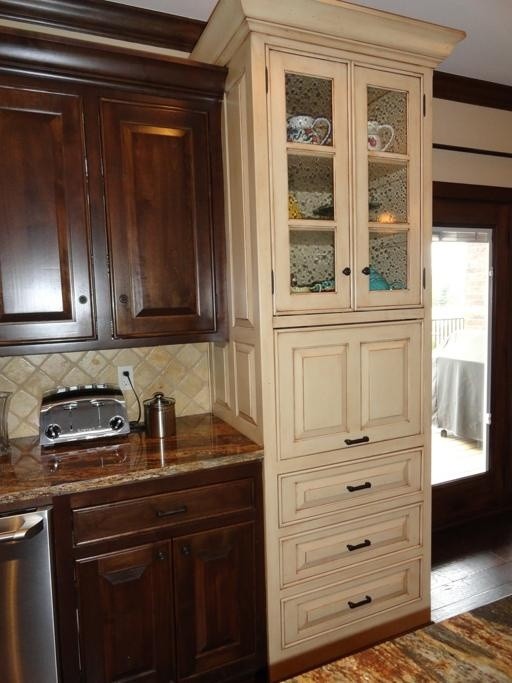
[39,383,131,449]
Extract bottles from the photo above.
[143,392,178,440]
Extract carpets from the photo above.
[280,595,512,682]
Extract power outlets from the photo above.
[118,365,134,391]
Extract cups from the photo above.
[367,120,394,151]
[289,114,330,144]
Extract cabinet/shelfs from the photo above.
[1,24,227,357]
[54,456,268,682]
[188,1,466,683]
